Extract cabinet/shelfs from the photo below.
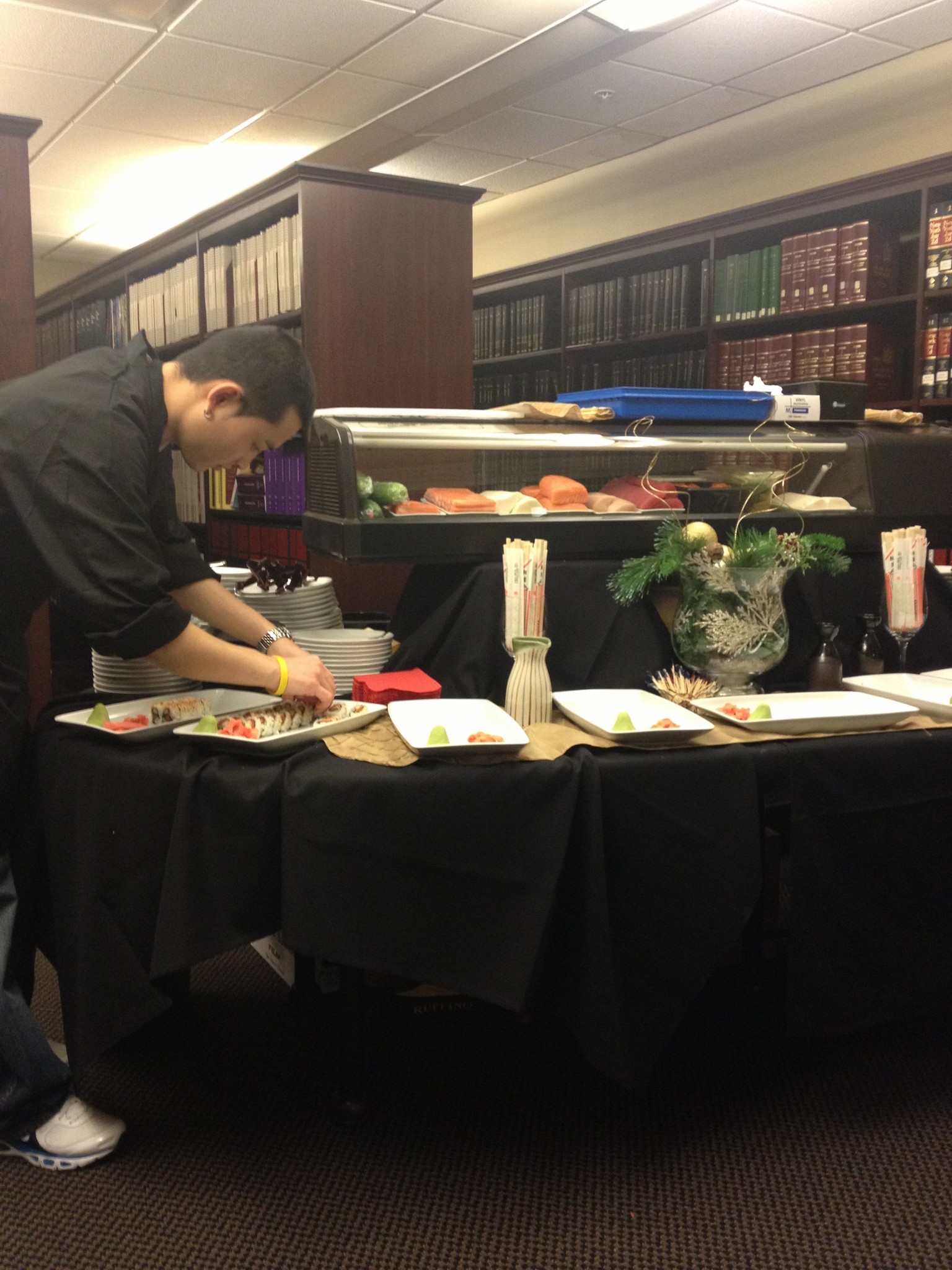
[0,106,45,728]
[34,162,486,573]
[467,150,952,424]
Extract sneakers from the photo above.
[0,1093,124,1171]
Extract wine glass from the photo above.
[879,578,929,673]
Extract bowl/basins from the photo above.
[647,677,723,714]
[674,464,787,513]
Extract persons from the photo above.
[0,322,336,1169]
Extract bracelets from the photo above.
[266,655,288,696]
[257,626,294,654]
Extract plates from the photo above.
[770,497,857,515]
[54,688,283,740]
[841,673,952,719]
[688,692,921,735]
[552,687,716,744]
[89,559,394,695]
[380,497,686,517]
[171,699,387,752]
[387,698,530,757]
[919,668,952,681]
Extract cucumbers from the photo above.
[355,470,409,521]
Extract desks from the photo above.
[13,657,952,1173]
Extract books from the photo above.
[38,215,308,576]
[474,199,951,410]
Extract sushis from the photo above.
[217,697,368,739]
[150,696,213,725]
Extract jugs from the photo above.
[505,637,553,730]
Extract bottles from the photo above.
[805,621,842,691]
[856,613,884,675]
